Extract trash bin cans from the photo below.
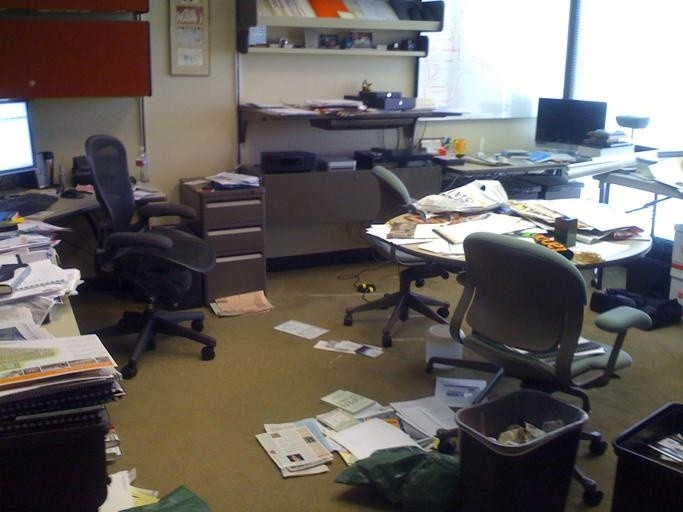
[610,402,683,512]
[454,388,589,512]
[150,223,202,311]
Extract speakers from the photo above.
[21,152,48,188]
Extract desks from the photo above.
[0,175,168,512]
[371,199,653,269]
[442,152,584,199]
[592,149,683,290]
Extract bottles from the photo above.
[135,146,148,185]
[58,160,66,185]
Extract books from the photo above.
[99,472,159,512]
[513,337,605,360]
[364,178,650,259]
[266,1,399,20]
[253,377,493,479]
[105,432,122,462]
[571,128,636,165]
[528,151,551,163]
[201,171,260,188]
[648,432,682,464]
[0,209,24,237]
[206,287,274,317]
[502,150,529,159]
[1,370,125,433]
[307,98,364,108]
[0,260,66,324]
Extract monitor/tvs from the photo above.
[534,97,605,154]
[0,97,35,178]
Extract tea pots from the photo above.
[38,152,52,186]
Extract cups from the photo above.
[453,138,465,158]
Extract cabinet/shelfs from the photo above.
[235,0,462,259]
[180,177,268,308]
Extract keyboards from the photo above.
[1,192,56,218]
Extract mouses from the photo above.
[61,189,82,198]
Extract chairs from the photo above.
[344,165,451,347]
[86,134,217,380]
[434,232,653,456]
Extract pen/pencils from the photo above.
[432,229,455,245]
